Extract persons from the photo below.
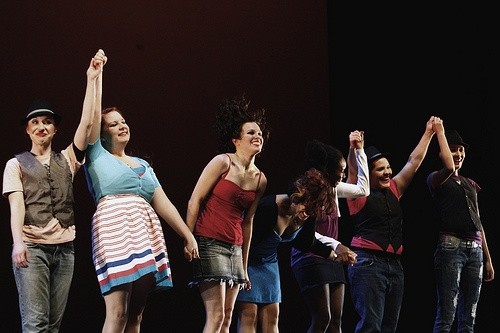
[433,116,494,333]
[236,168,336,333]
[345,116,436,333]
[84,49,200,333]
[3,57,101,333]
[290,131,370,333]
[184,95,268,333]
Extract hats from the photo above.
[26,100,55,119]
[446,130,468,149]
[365,146,388,160]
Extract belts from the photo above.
[440,235,480,249]
